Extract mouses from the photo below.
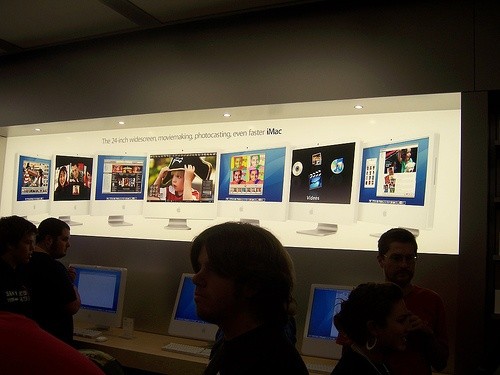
[96,337,108,342]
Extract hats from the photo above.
[162,156,213,185]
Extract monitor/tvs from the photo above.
[168,272,218,341]
[285,139,359,237]
[69,263,128,329]
[216,145,287,226]
[357,133,439,238]
[301,283,354,359]
[11,152,53,223]
[50,155,96,226]
[143,152,218,231]
[92,155,146,226]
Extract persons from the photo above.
[153,156,212,201]
[16,218,81,349]
[56,164,92,195]
[249,155,265,170]
[0,217,42,325]
[231,170,246,184]
[336,230,450,375]
[190,225,310,375]
[248,170,263,184]
[231,157,247,170]
[330,283,414,375]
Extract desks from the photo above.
[73,320,340,375]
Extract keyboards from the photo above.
[161,343,212,358]
[73,328,102,338]
[305,364,334,373]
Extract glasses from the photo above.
[380,251,417,263]
